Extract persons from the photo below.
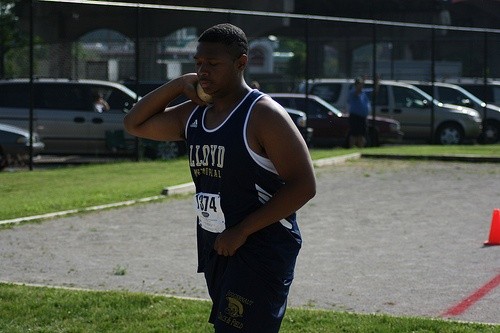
[347,69,378,147]
[122,23,316,333]
[93,92,110,112]
[250,81,260,91]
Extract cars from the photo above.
[264,93,403,148]
[0,123,45,172]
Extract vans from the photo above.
[0,79,174,159]
[395,79,500,145]
[299,78,483,145]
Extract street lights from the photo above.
[422,5,452,145]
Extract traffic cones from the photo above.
[483,208,500,246]
